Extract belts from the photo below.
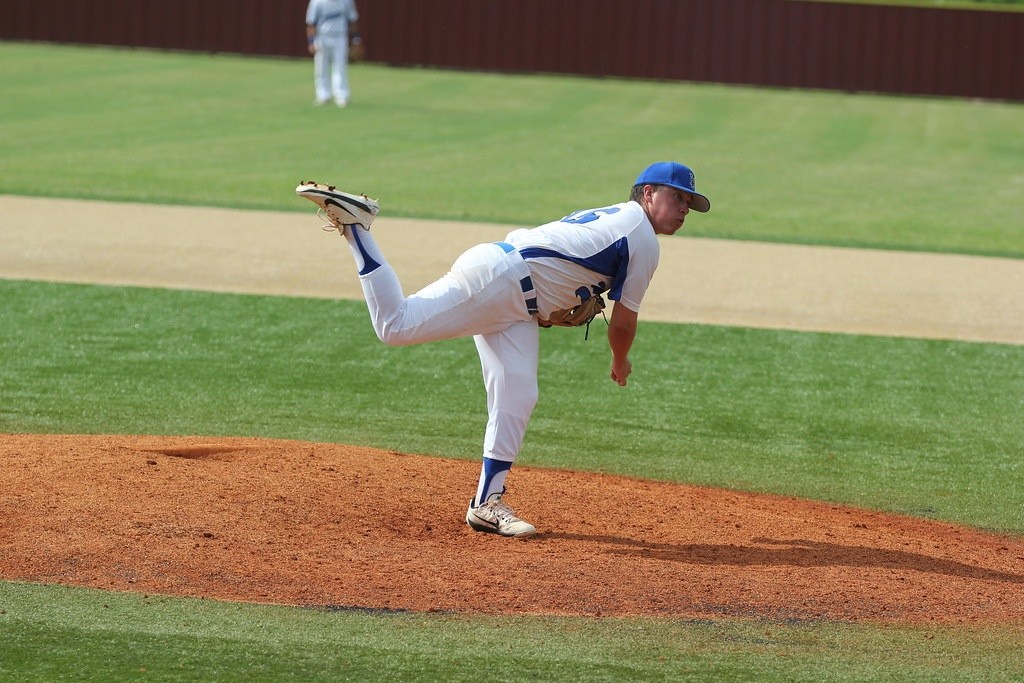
[494,242,538,314]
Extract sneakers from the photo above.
[465,486,536,538]
[296,180,379,236]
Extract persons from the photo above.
[295,161,710,537]
[305,0,362,109]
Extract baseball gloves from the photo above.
[538,294,606,329]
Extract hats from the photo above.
[634,162,710,213]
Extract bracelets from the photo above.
[308,37,314,44]
